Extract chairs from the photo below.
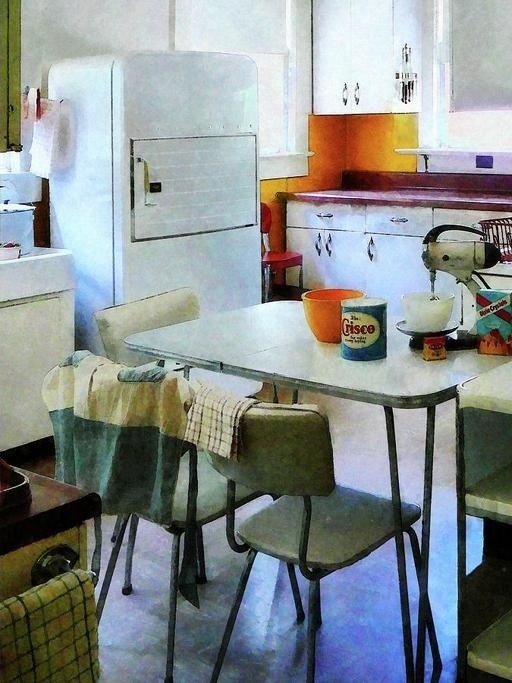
[63,349,306,683]
[92,285,261,596]
[188,376,444,683]
[260,202,303,303]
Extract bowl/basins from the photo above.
[402,291,455,332]
[302,290,368,344]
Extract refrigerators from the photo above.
[49,50,265,360]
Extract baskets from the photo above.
[478,217,512,265]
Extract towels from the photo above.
[0,569,102,683]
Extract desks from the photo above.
[122,300,511,682]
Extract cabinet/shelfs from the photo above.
[312,0,422,116]
[456,360,512,683]
[275,171,512,327]
